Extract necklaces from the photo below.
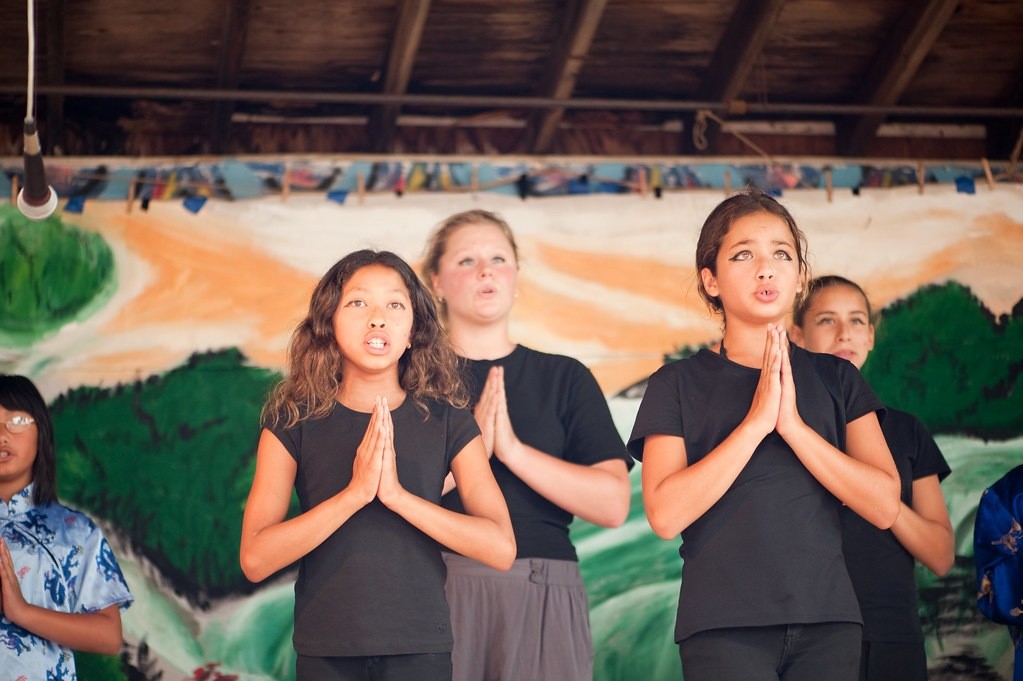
[720,338,728,359]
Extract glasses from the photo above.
[0,414,37,434]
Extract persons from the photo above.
[241,250,517,679]
[0,374,131,681]
[420,209,634,679]
[625,193,901,681]
[789,275,955,681]
